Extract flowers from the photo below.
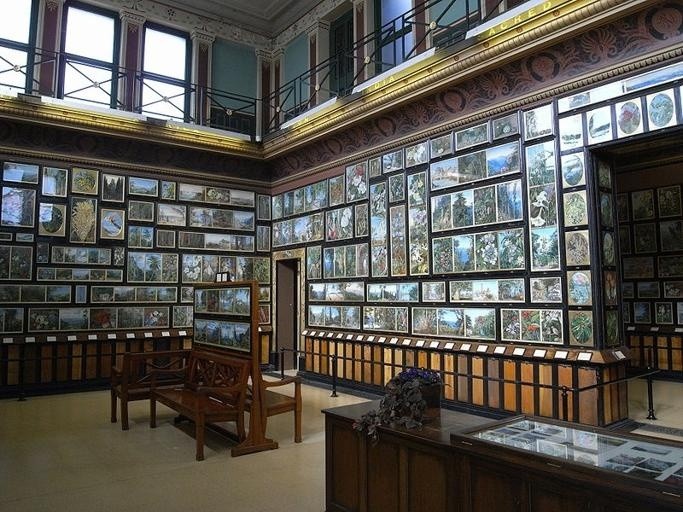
[352,366,453,449]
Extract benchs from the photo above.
[108,344,304,462]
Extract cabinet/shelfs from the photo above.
[319,407,683,512]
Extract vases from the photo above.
[421,390,441,408]
